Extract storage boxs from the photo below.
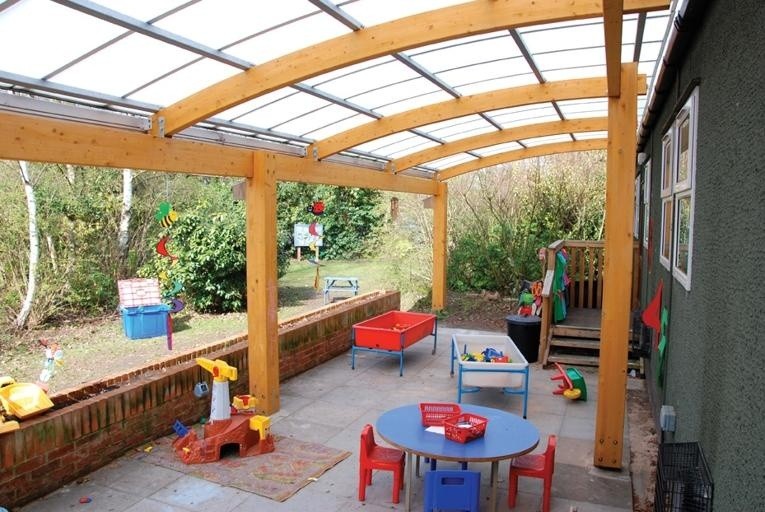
[351,309,437,352]
[450,333,529,391]
[117,276,172,339]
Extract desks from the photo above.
[375,402,541,512]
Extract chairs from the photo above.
[423,470,481,512]
[507,433,557,512]
[358,423,406,505]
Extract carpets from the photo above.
[124,424,353,504]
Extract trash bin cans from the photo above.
[505,316,542,363]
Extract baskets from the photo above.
[444,411,488,443]
[653,443,712,511]
[418,401,461,426]
